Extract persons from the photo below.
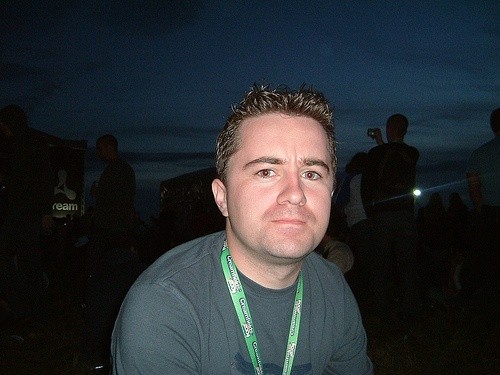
[0,104,53,333]
[110,85,372,375]
[316,108,500,333]
[90,135,135,338]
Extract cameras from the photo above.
[368,129,375,136]
